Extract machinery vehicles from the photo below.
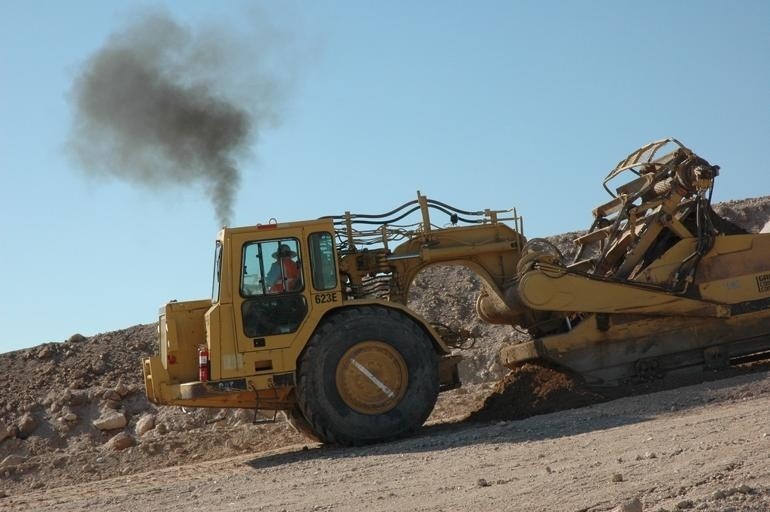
[140,136,769,448]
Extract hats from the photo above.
[272,245,297,258]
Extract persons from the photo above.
[265,244,302,292]
